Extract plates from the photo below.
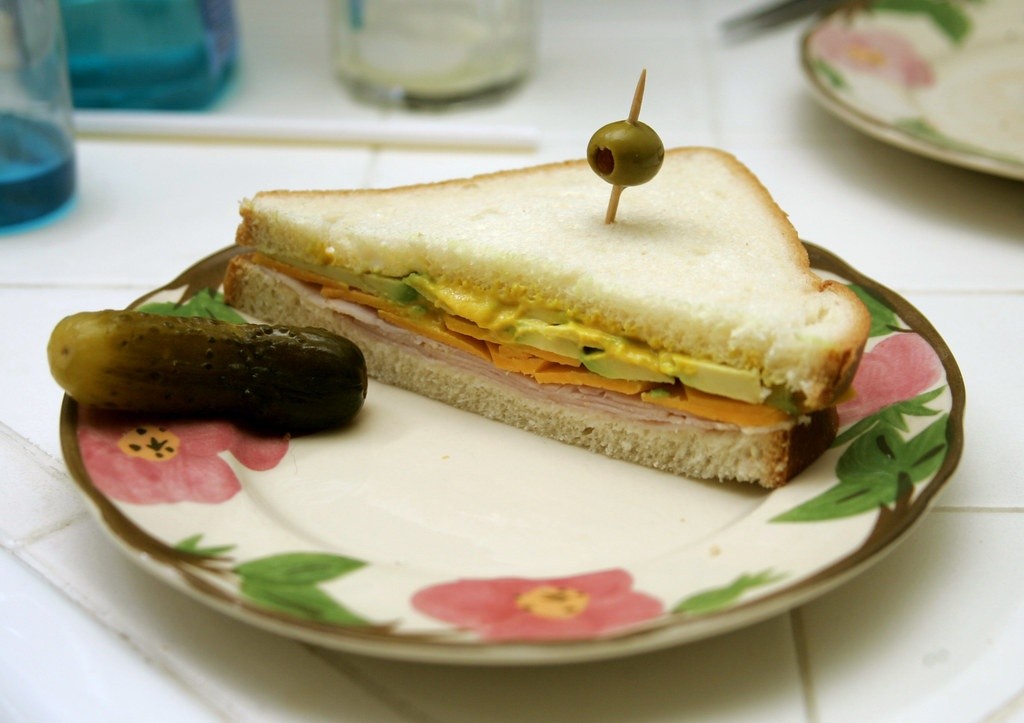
[56,239,966,670]
[798,0,1024,189]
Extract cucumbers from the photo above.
[46,309,367,432]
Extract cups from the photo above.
[0,3,77,238]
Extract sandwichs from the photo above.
[223,146,873,490]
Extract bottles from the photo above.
[10,0,240,115]
[327,0,540,111]
